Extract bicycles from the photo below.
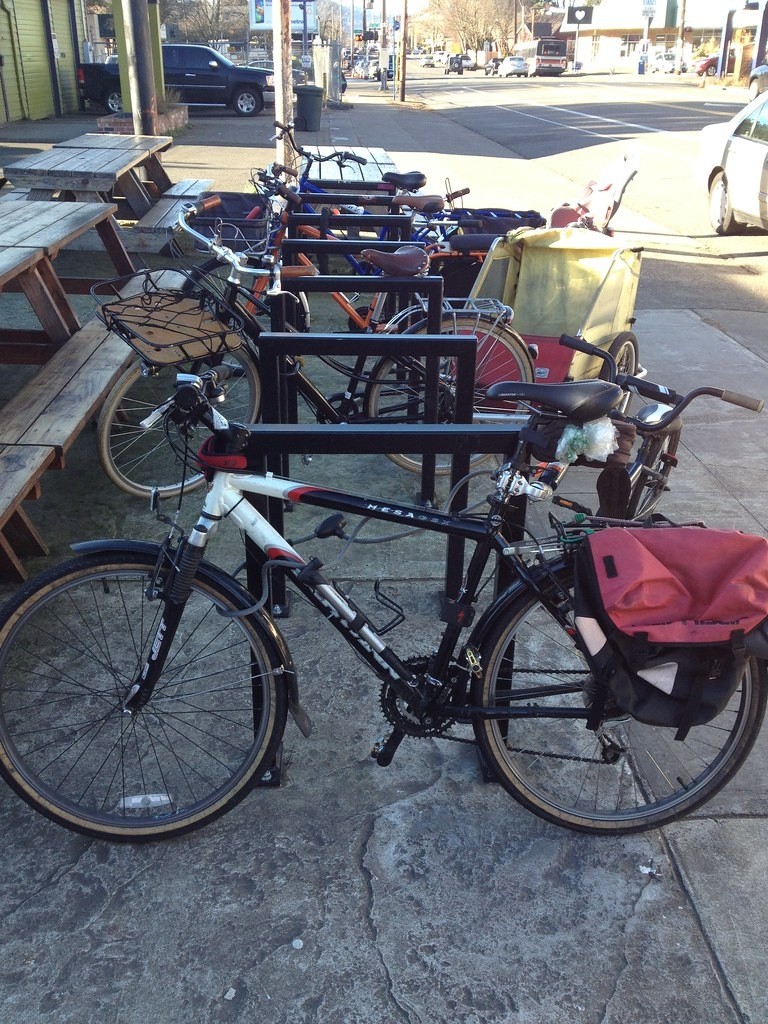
[0,359,768,843]
[176,117,550,385]
[555,332,768,587]
[87,196,538,499]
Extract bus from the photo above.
[512,37,567,76]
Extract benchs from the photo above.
[0,270,192,583]
[0,447,56,532]
[134,201,197,235]
[161,177,217,200]
[0,187,30,202]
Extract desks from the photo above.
[0,201,136,415]
[4,133,186,259]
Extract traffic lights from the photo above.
[355,34,364,41]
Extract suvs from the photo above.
[444,57,463,75]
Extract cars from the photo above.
[76,43,298,115]
[749,52,768,101]
[432,50,479,70]
[694,44,758,77]
[421,55,435,69]
[246,61,309,85]
[499,55,528,78]
[697,90,768,238]
[341,49,399,80]
[484,56,504,75]
